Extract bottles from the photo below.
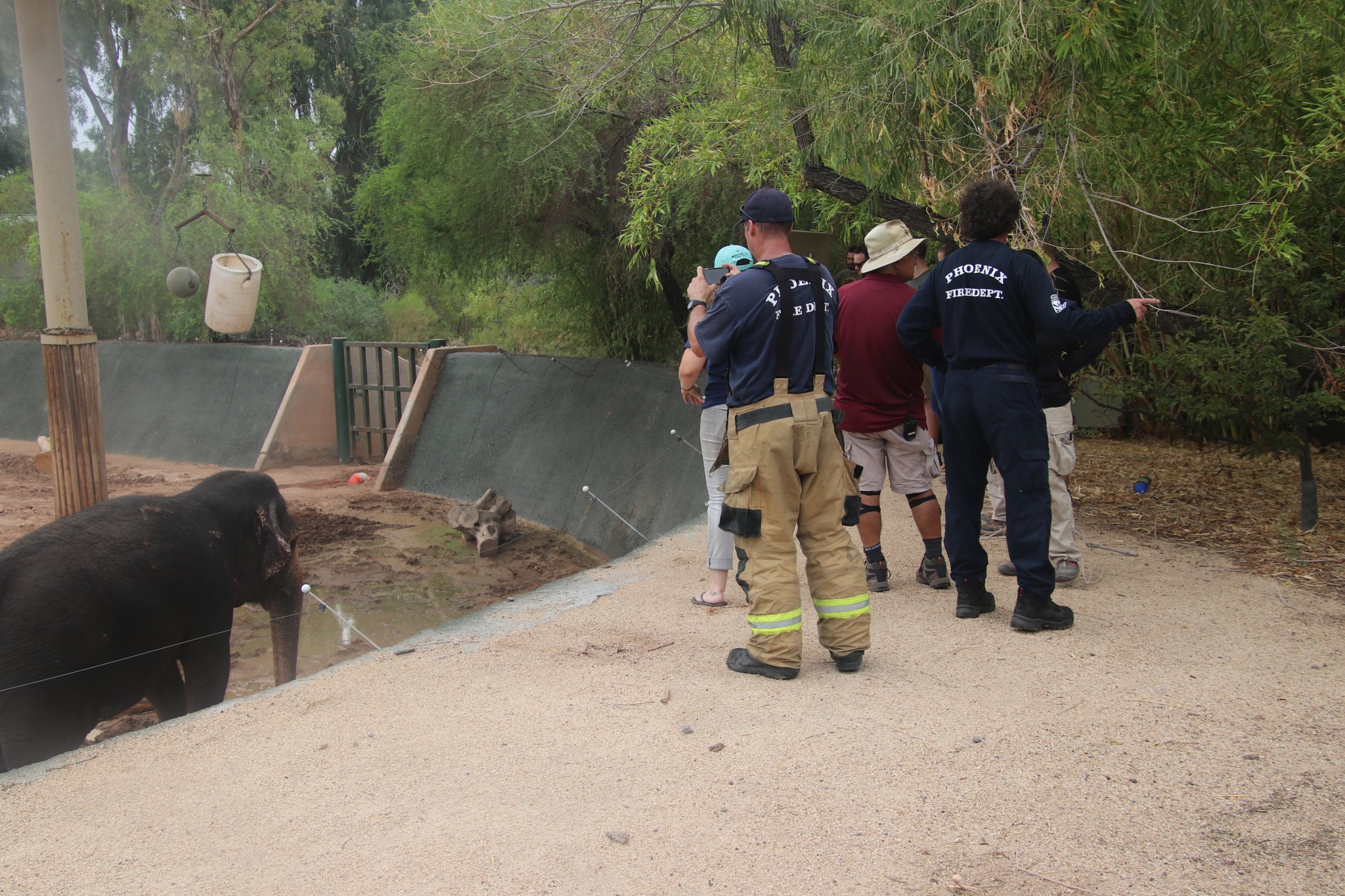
[1133,475,1152,493]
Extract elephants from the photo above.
[0,470,305,773]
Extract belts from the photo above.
[982,360,1029,371]
[736,397,835,431]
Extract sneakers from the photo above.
[865,556,891,592]
[956,579,995,618]
[998,562,1017,575]
[1010,587,1074,631]
[1056,560,1080,582]
[980,512,1001,525]
[916,553,950,589]
[980,521,1007,536]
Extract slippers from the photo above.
[691,592,727,606]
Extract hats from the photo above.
[734,187,793,228]
[860,220,931,273]
[714,244,753,272]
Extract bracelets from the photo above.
[681,384,694,390]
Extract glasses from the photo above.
[909,250,919,260]
[739,206,755,223]
[855,261,866,268]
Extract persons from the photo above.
[896,178,1161,632]
[687,186,871,680]
[835,219,1113,591]
[678,244,753,607]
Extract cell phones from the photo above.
[702,266,730,285]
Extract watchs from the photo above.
[687,299,707,312]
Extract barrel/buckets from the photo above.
[204,252,262,335]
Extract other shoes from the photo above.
[829,650,865,671]
[726,648,801,679]
